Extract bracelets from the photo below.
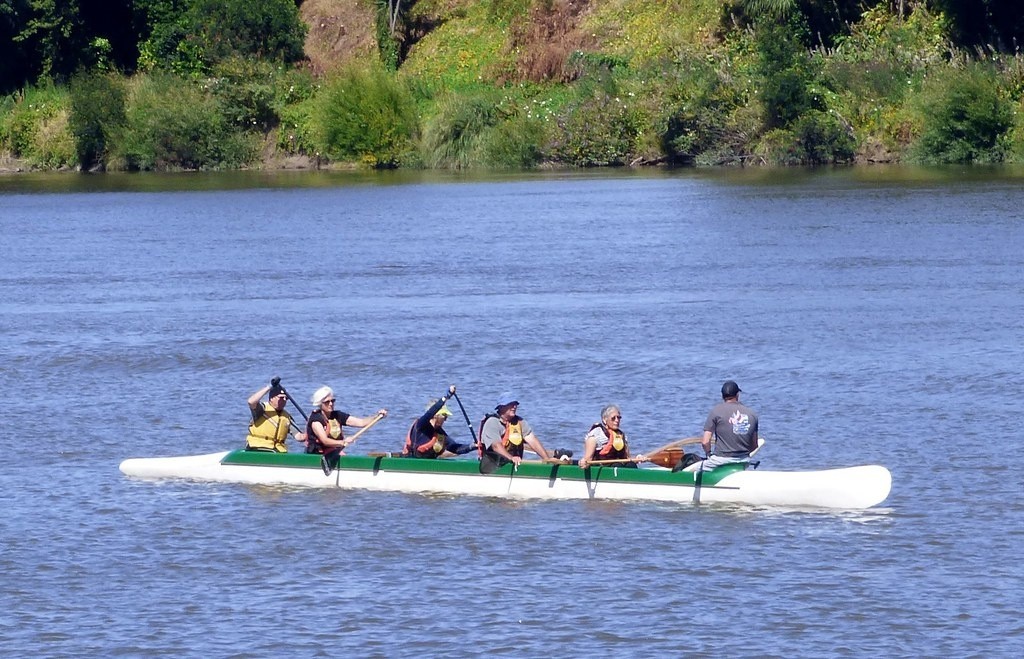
[444,390,452,399]
[342,440,344,447]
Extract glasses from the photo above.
[275,395,288,400]
[605,416,621,421]
[322,399,335,405]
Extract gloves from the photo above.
[271,377,281,388]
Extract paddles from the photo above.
[278,382,309,422]
[521,458,580,466]
[453,392,479,443]
[320,413,384,476]
[585,448,685,469]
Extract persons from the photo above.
[304,386,387,456]
[477,391,558,469]
[578,402,647,470]
[246,383,307,453]
[680,380,759,473]
[401,384,478,459]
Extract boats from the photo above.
[116,447,894,511]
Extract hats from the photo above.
[722,382,742,397]
[269,386,285,401]
[425,402,453,416]
[494,392,520,410]
[313,388,330,406]
